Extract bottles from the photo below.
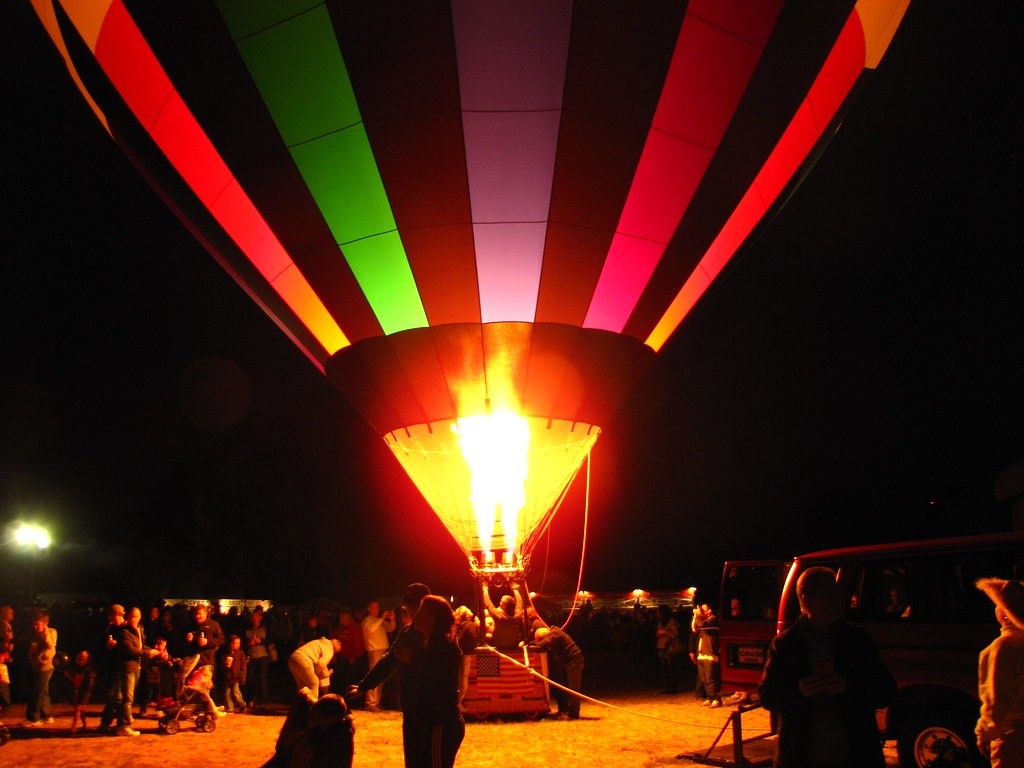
[200,632,204,638]
[163,649,167,653]
[253,634,256,639]
[108,634,113,649]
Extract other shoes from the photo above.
[116,726,141,736]
[703,700,710,706]
[20,719,43,725]
[43,717,54,724]
[141,701,257,720]
[710,699,721,707]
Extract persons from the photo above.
[242,583,465,768]
[759,566,885,768]
[455,572,585,721]
[0,603,246,737]
[974,575,1024,768]
[546,597,723,709]
[886,582,911,617]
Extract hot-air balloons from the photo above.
[25,0,916,721]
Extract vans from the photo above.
[717,532,1024,768]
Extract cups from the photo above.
[225,657,233,668]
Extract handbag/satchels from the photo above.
[667,631,684,654]
[266,641,277,661]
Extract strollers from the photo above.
[152,652,223,732]
